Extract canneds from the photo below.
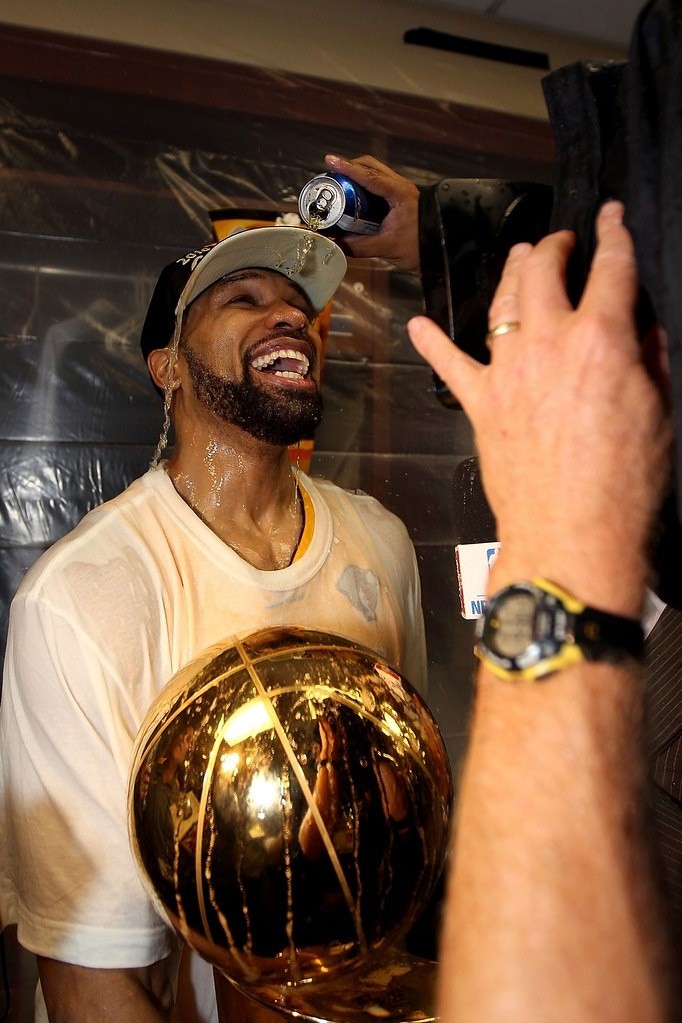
[299,170,390,237]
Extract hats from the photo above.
[139,226,348,404]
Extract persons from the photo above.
[325,0,682,616]
[406,199,682,1023]
[0,226,429,1023]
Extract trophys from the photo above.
[128,625,455,1023]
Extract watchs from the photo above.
[471,576,648,684]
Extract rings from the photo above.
[485,319,519,347]
